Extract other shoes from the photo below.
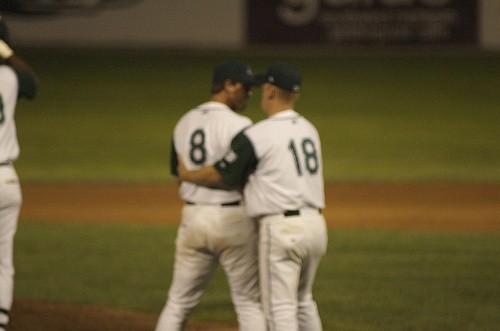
[0,308,9,331]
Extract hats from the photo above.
[253,60,302,93]
[212,62,263,86]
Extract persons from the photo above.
[154,62,269,331]
[177,61,328,331]
[0,18,39,331]
